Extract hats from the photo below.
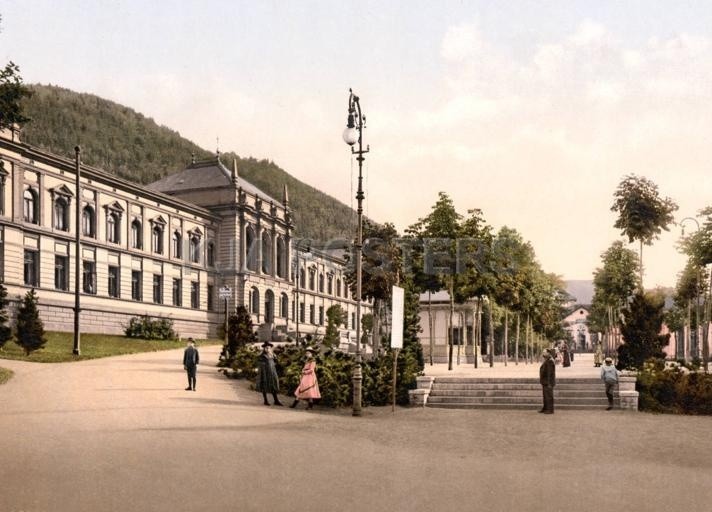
[262,341,273,347]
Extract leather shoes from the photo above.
[186,386,196,391]
[264,400,312,409]
[538,409,554,414]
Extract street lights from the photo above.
[293,236,313,345]
[339,85,373,419]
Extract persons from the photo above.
[182,336,200,391]
[536,339,620,415]
[287,346,322,409]
[255,340,284,406]
